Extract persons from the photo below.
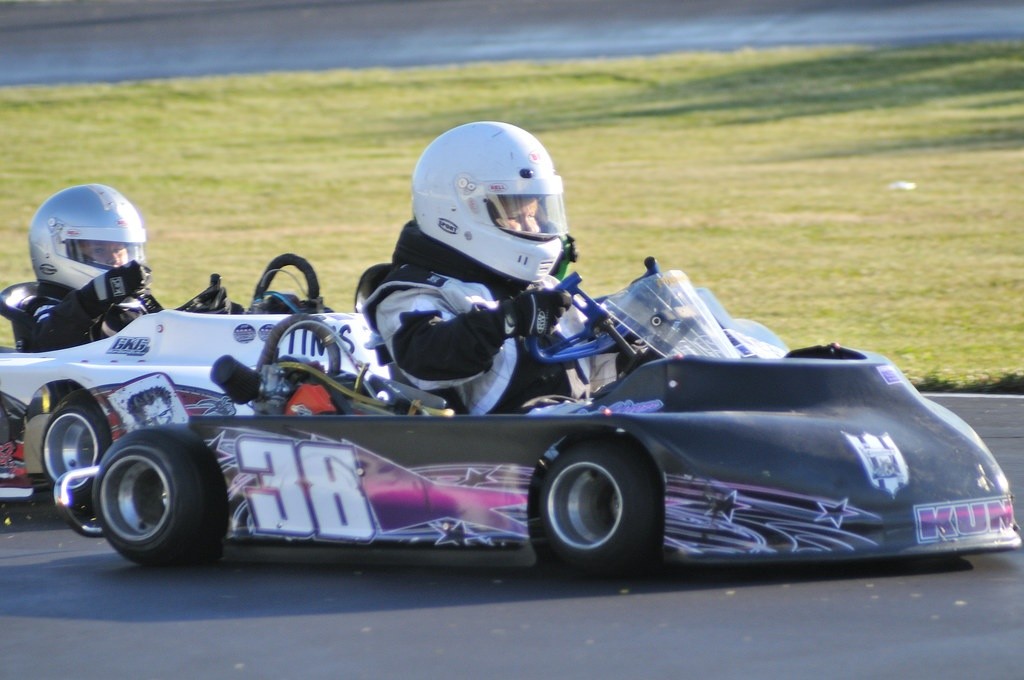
[361,120,683,414]
[17,184,150,352]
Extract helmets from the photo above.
[28,183,146,290]
[410,122,568,284]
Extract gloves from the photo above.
[78,259,151,318]
[501,288,572,339]
[186,284,232,315]
